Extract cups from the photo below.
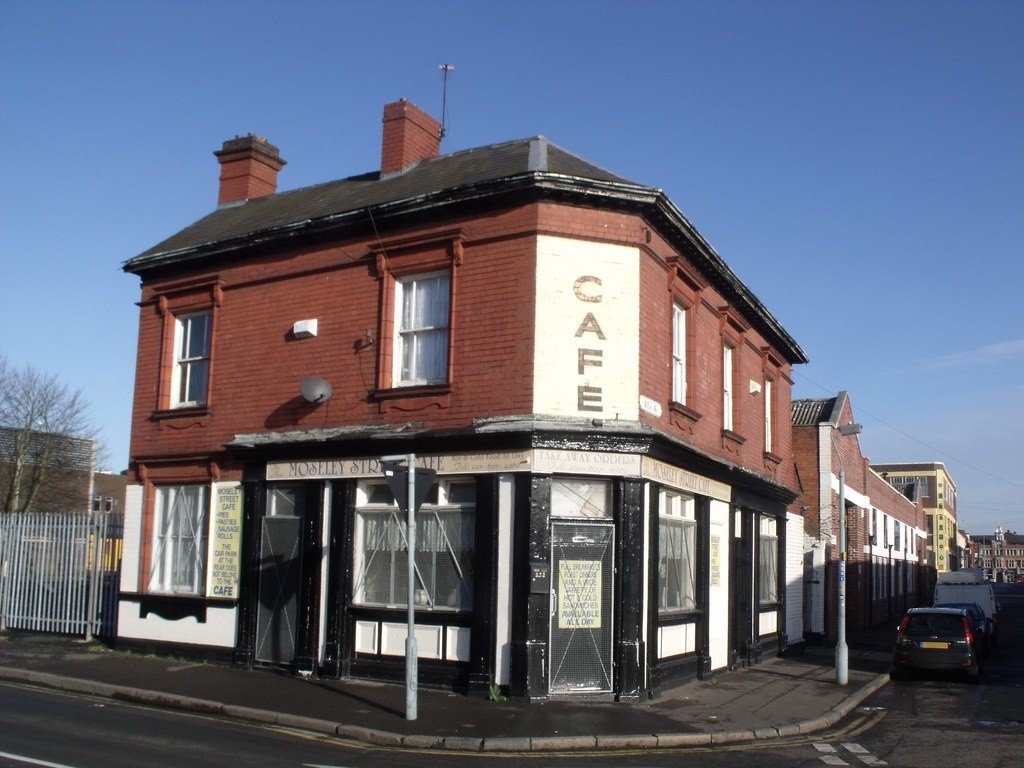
[415,590,427,605]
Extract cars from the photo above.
[892,606,982,685]
[931,603,994,659]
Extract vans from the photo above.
[931,571,1002,646]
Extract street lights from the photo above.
[830,422,864,684]
[379,452,420,721]
